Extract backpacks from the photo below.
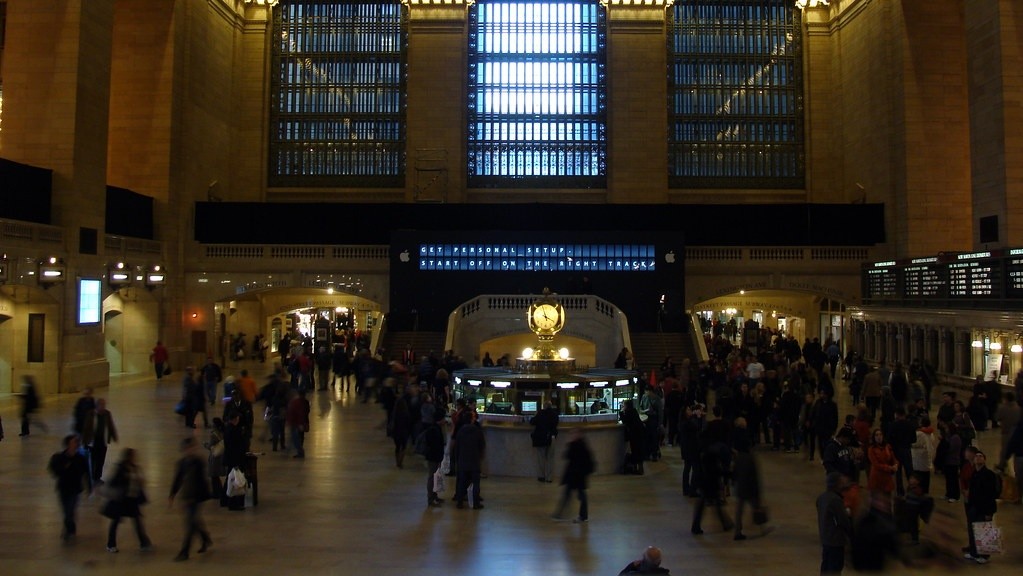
[414,426,439,455]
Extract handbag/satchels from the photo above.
[210,432,227,458]
[431,452,451,492]
[226,467,248,497]
[972,521,1004,555]
[969,439,980,451]
[174,399,190,416]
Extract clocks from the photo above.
[533,304,558,329]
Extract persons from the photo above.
[201,357,222,405]
[844,475,870,528]
[867,429,898,496]
[168,438,213,561]
[202,314,1023,541]
[48,434,88,544]
[528,399,558,485]
[81,399,118,485]
[74,387,94,435]
[959,447,978,497]
[16,375,38,437]
[100,447,158,553]
[961,473,1002,553]
[176,365,199,426]
[149,342,168,381]
[964,453,997,563]
[850,496,913,576]
[816,472,854,576]
[192,368,211,428]
[619,545,672,576]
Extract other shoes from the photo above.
[948,498,960,503]
[940,496,948,499]
[964,554,974,559]
[976,556,991,563]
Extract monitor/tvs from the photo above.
[76,274,103,327]
